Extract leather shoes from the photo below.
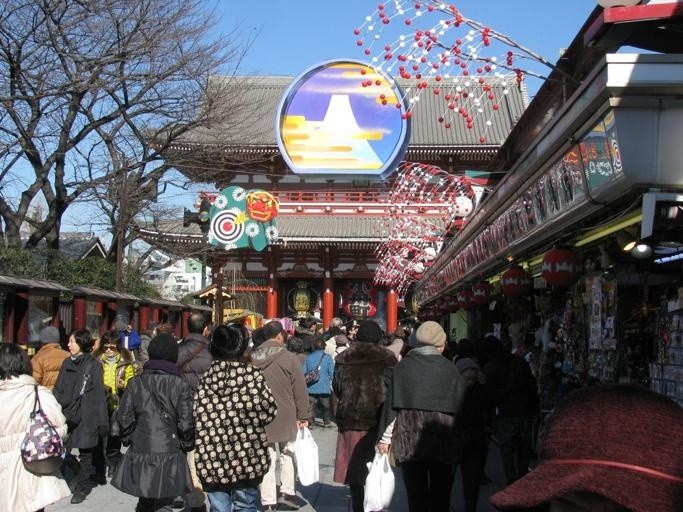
[108,464,116,477]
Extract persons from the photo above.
[332,319,542,511]
[116,314,359,511]
[1,313,156,512]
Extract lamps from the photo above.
[574,209,642,248]
[529,252,544,266]
[483,260,526,285]
[632,245,653,259]
[616,230,636,252]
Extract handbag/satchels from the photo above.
[362,446,397,512]
[19,408,65,464]
[288,424,320,487]
[303,368,320,388]
[63,398,83,432]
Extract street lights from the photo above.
[179,198,211,289]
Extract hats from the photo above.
[207,321,250,359]
[39,326,61,345]
[112,319,128,332]
[355,319,382,343]
[456,358,480,374]
[415,320,447,350]
[147,333,179,364]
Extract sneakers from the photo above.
[70,493,85,504]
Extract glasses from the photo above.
[104,345,117,352]
